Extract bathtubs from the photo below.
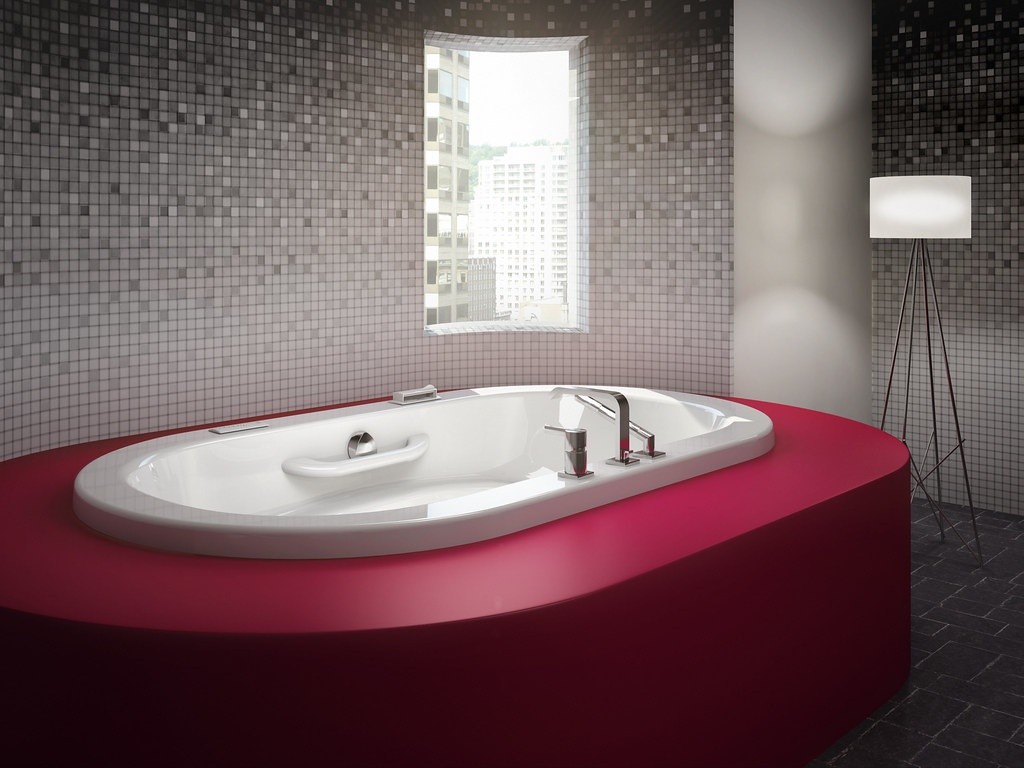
[67,381,774,562]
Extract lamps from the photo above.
[869,174,983,569]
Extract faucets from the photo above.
[572,388,668,462]
[547,385,642,469]
